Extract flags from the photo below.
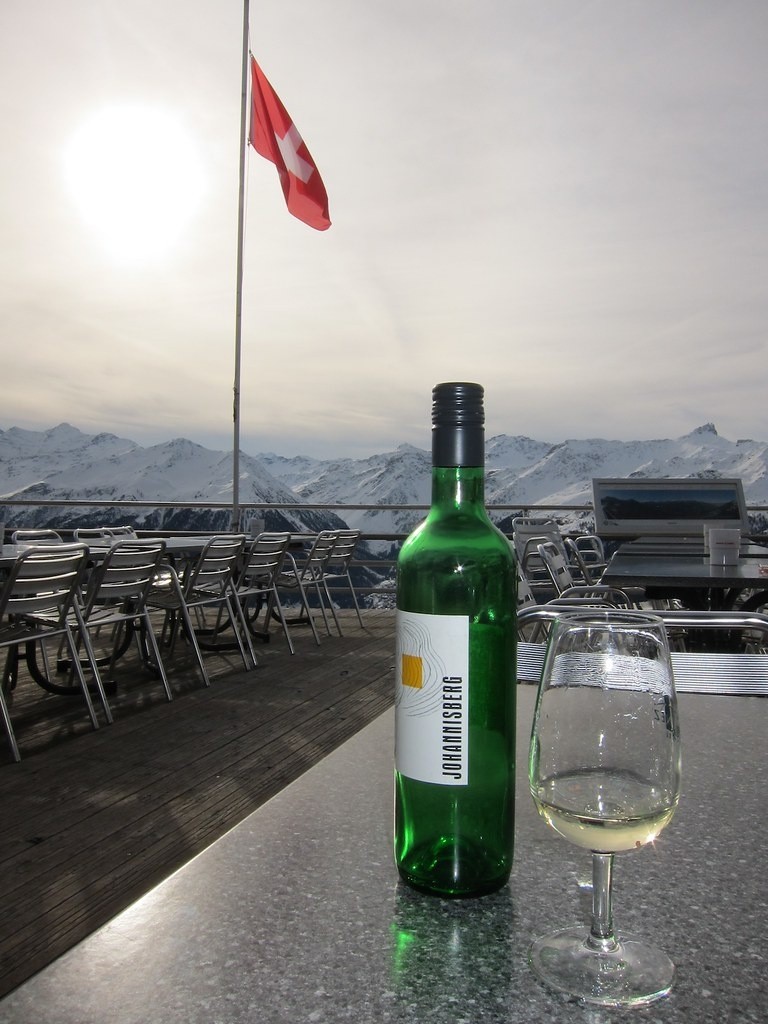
[250,55,332,231]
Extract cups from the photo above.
[757,560,768,577]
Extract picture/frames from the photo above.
[591,478,751,537]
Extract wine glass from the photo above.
[529,610,680,1007]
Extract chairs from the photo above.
[0,516,691,764]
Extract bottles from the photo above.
[397,382,520,898]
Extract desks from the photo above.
[600,536,768,653]
[0,535,316,696]
[0,682,768,1024]
